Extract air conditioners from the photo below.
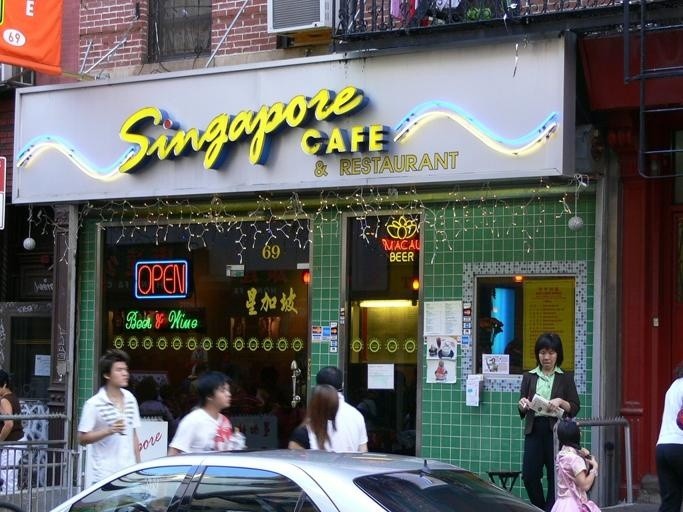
[266,0,340,36]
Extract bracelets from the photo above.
[589,469,598,478]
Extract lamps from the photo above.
[23,207,36,250]
[569,173,583,231]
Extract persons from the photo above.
[77,348,142,490]
[288,384,350,450]
[315,365,368,451]
[167,370,236,457]
[0,368,25,493]
[653,364,683,512]
[517,331,581,512]
[549,419,604,512]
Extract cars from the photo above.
[41,440,561,512]
